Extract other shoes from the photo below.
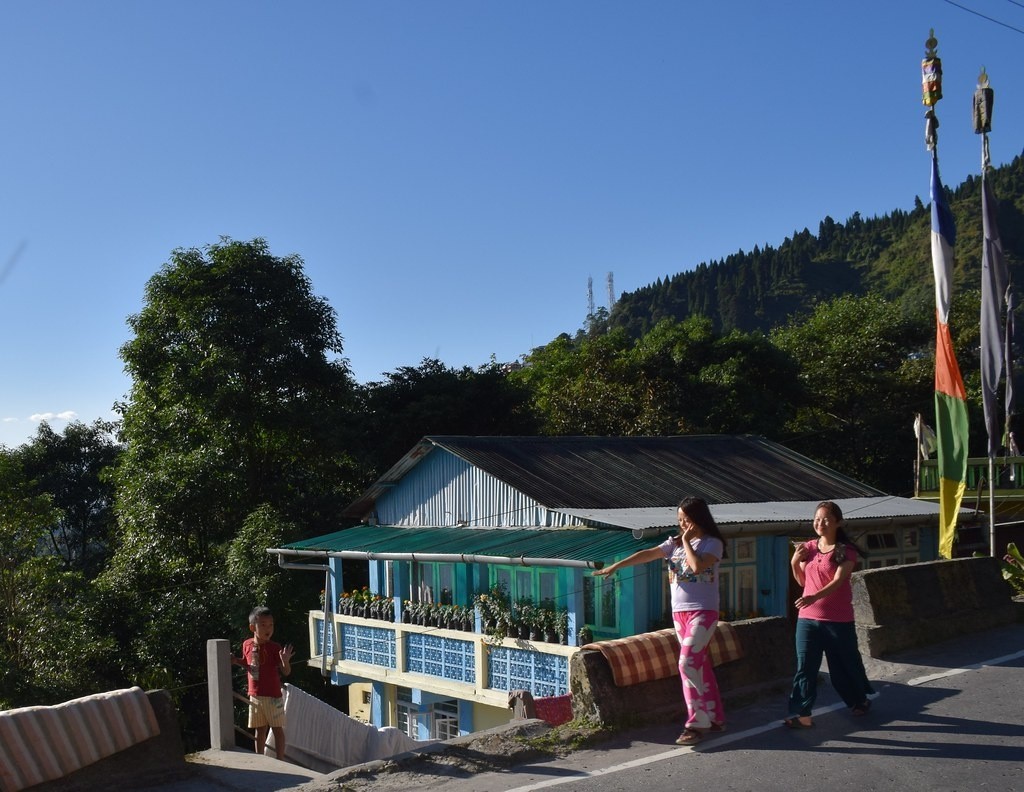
[708,721,726,733]
[675,727,705,744]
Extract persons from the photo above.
[785,501,876,731]
[591,496,729,745]
[230,606,296,761]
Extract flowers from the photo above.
[320,582,553,655]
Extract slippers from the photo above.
[782,716,816,729]
[853,699,871,718]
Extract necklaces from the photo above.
[817,539,835,562]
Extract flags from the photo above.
[914,418,938,460]
[979,168,1018,460]
[928,154,970,559]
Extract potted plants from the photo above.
[554,606,569,645]
[576,626,592,647]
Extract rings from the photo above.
[800,597,804,601]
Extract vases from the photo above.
[322,605,553,643]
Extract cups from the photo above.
[792,540,807,562]
